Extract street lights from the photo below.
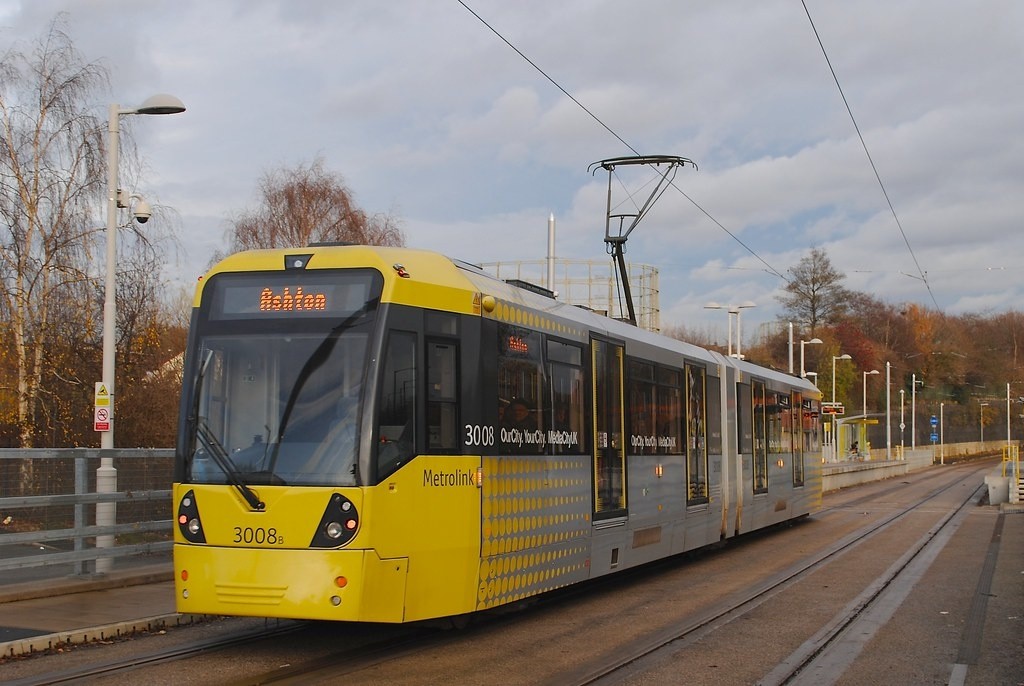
[800,339,823,383]
[703,300,759,362]
[830,353,949,465]
[95,92,186,576]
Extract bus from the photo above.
[170,239,824,632]
